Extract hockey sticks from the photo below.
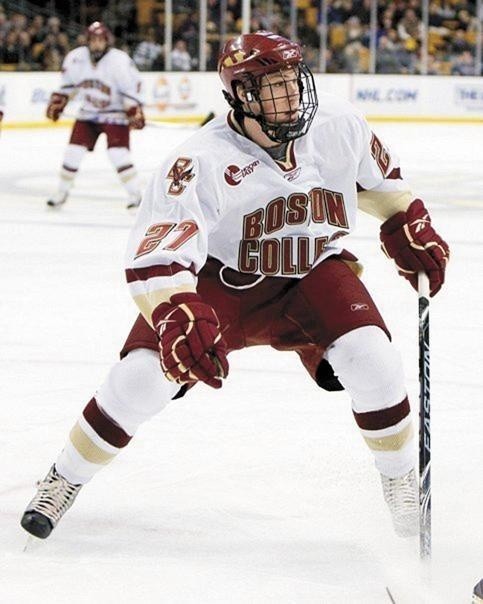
[55,112,214,131]
[385,271,430,604]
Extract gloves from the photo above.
[127,105,144,129]
[47,93,69,120]
[152,293,229,388]
[380,199,449,296]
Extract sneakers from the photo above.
[21,464,81,539]
[48,194,65,206]
[381,470,419,524]
[127,194,141,207]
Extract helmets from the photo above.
[218,30,318,143]
[86,22,111,43]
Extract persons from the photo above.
[45,21,146,209]
[20,29,449,540]
